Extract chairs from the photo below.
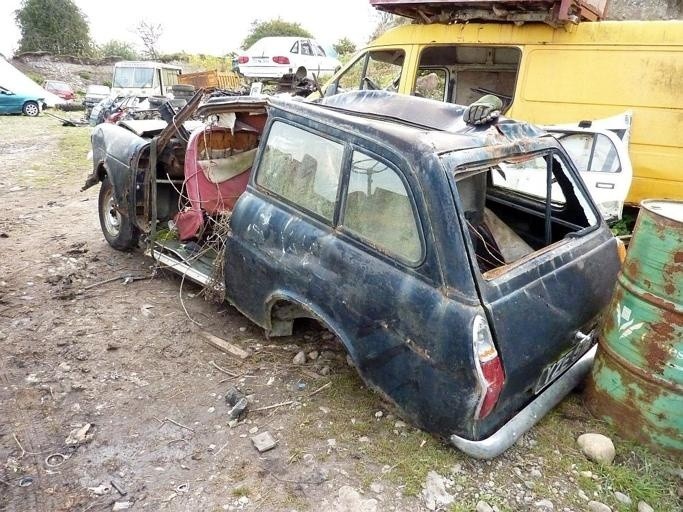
[172,120,259,241]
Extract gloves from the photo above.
[462,93,504,127]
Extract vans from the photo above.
[305,19,683,208]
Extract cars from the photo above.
[0,86,47,116]
[238,36,342,79]
[86,86,111,108]
[40,80,76,99]
[82,89,625,460]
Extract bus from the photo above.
[111,61,182,103]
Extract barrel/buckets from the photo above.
[582,198,683,462]
